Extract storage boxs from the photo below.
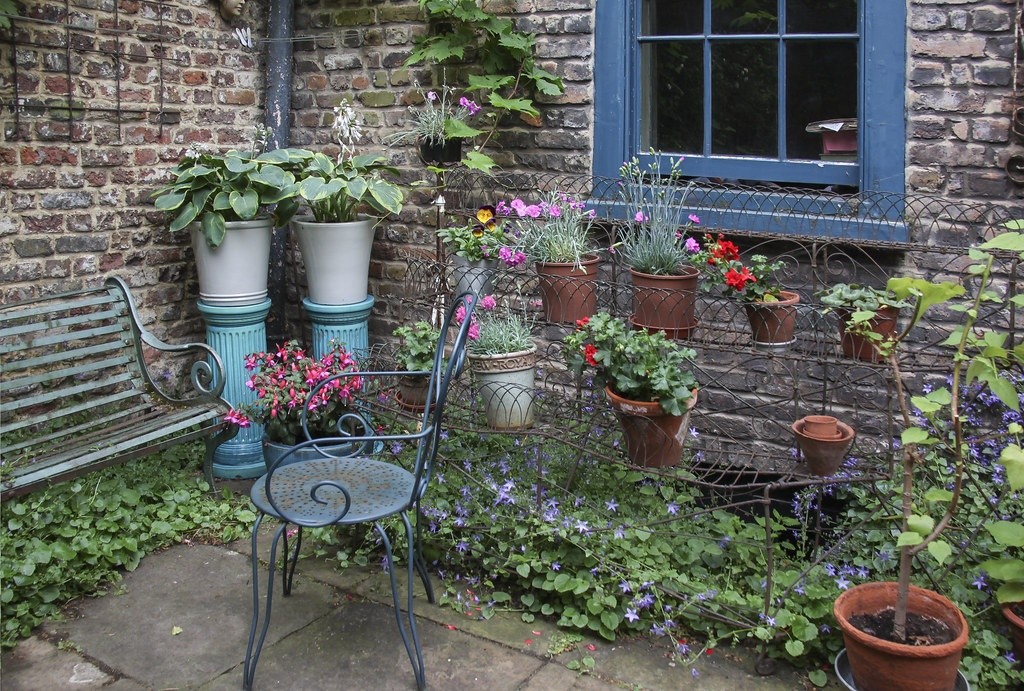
[821,131,857,154]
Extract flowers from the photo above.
[381,79,482,146]
[222,338,389,448]
[150,97,315,249]
[391,148,786,416]
[275,98,403,229]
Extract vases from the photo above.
[604,383,697,466]
[451,254,498,299]
[292,213,378,305]
[628,265,701,327]
[536,255,599,324]
[792,415,853,475]
[418,136,462,161]
[743,292,800,343]
[189,218,273,307]
[466,342,537,431]
[261,422,374,473]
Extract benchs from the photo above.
[0,275,239,497]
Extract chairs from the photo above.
[224,291,479,691]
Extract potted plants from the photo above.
[392,320,457,406]
[814,215,1024,691]
